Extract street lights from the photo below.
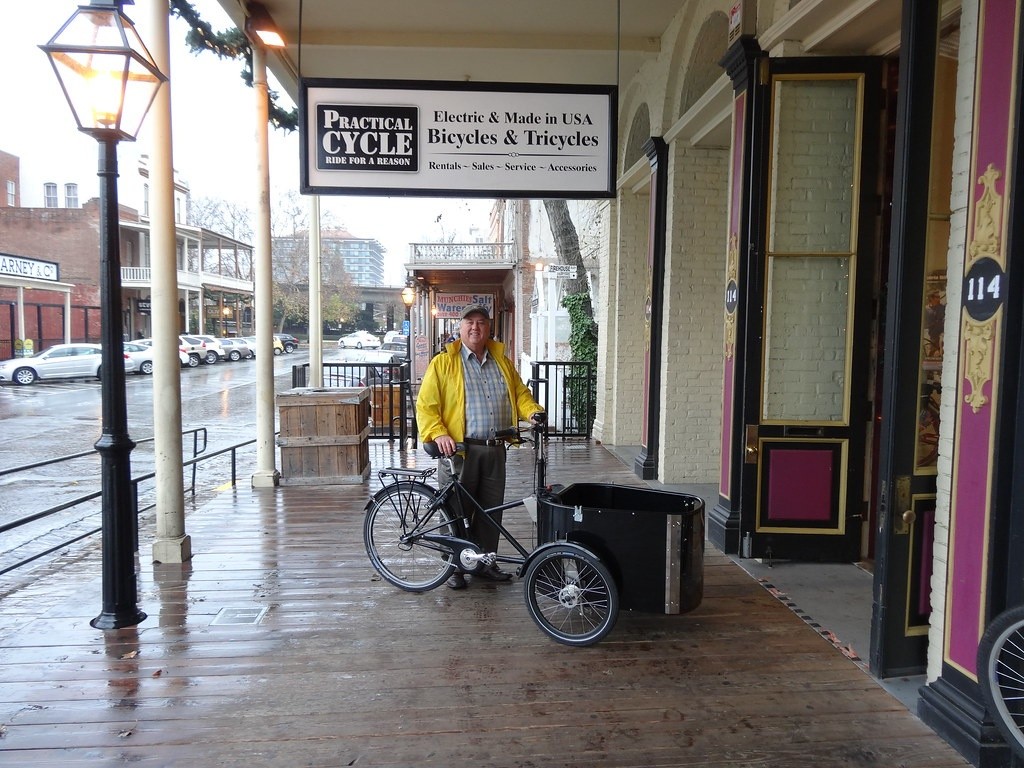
[431,301,438,355]
[401,272,416,382]
[38,0,168,630]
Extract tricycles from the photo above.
[363,413,705,647]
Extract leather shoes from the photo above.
[472,564,513,581]
[447,572,465,588]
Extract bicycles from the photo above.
[975,607,1024,761]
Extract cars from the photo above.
[338,330,381,349]
[321,330,407,388]
[84,333,300,375]
[0,344,136,386]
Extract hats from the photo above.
[459,305,490,319]
[444,337,455,343]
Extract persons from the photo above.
[416,305,548,589]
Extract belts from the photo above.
[464,437,503,446]
